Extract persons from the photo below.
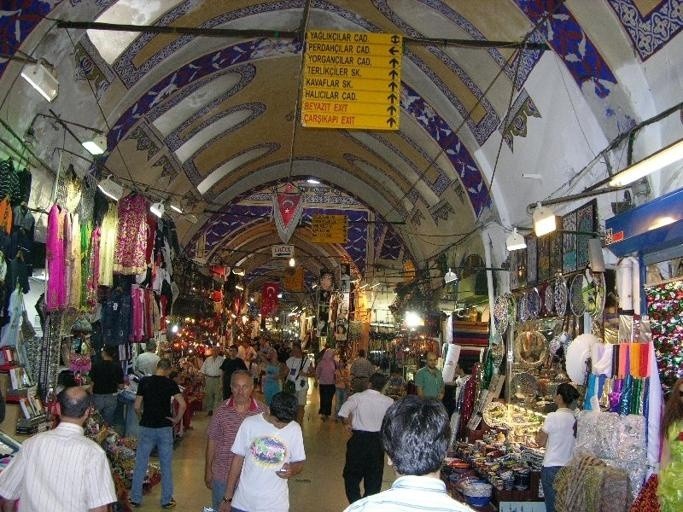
[127,358,187,511]
[44,369,80,403]
[337,324,346,341]
[337,372,394,504]
[339,309,348,317]
[244,339,257,376]
[85,344,125,427]
[350,349,374,394]
[333,358,351,422]
[315,346,336,422]
[197,346,226,417]
[233,339,247,368]
[263,349,285,408]
[169,371,181,384]
[218,391,306,512]
[316,320,326,337]
[285,342,315,426]
[537,384,576,511]
[218,344,248,403]
[0,386,117,512]
[454,360,474,410]
[340,263,349,280]
[343,395,477,512]
[204,368,270,511]
[416,352,445,400]
[133,338,161,376]
[252,335,269,394]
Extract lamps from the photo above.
[82,135,109,158]
[20,58,61,105]
[443,268,458,283]
[531,201,558,238]
[506,227,527,252]
[605,139,683,192]
[148,201,165,220]
[97,178,124,203]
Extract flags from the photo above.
[272,189,307,246]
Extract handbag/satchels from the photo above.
[338,367,351,395]
[284,355,309,395]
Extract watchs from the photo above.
[223,496,232,505]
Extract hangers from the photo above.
[3,153,34,177]
[132,280,160,293]
[50,200,83,219]
[3,194,34,218]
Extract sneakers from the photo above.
[128,495,140,509]
[335,418,339,424]
[162,498,176,509]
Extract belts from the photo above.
[207,374,221,379]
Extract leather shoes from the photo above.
[325,415,329,420]
[320,416,325,423]
[208,410,213,416]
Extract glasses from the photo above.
[229,349,237,352]
[427,359,438,362]
[678,390,683,398]
